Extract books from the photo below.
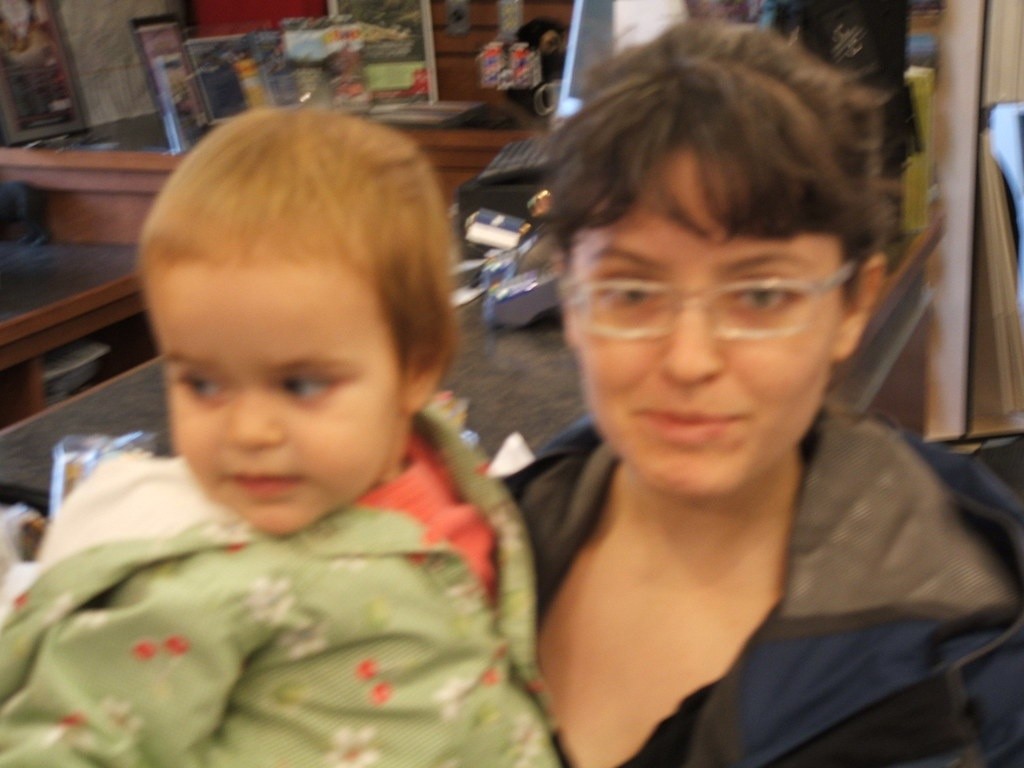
[130,0,439,157]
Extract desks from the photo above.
[0,106,550,246]
[0,239,146,427]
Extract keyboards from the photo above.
[479,135,553,183]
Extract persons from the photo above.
[0,103,561,767]
[770,0,927,242]
[493,18,1024,768]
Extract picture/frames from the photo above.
[129,15,372,153]
[0,0,92,144]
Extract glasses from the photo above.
[555,257,858,339]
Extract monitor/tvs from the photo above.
[558,0,616,115]
